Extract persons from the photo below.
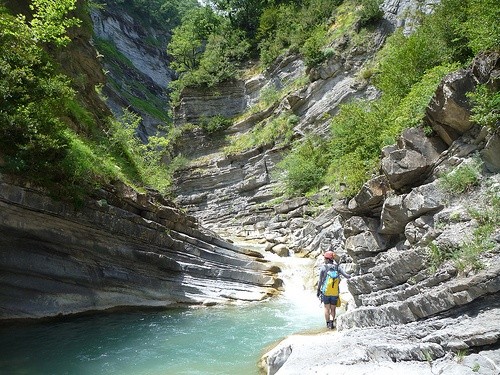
[316,251,352,329]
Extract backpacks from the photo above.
[321,264,341,297]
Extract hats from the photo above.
[324,251,336,260]
